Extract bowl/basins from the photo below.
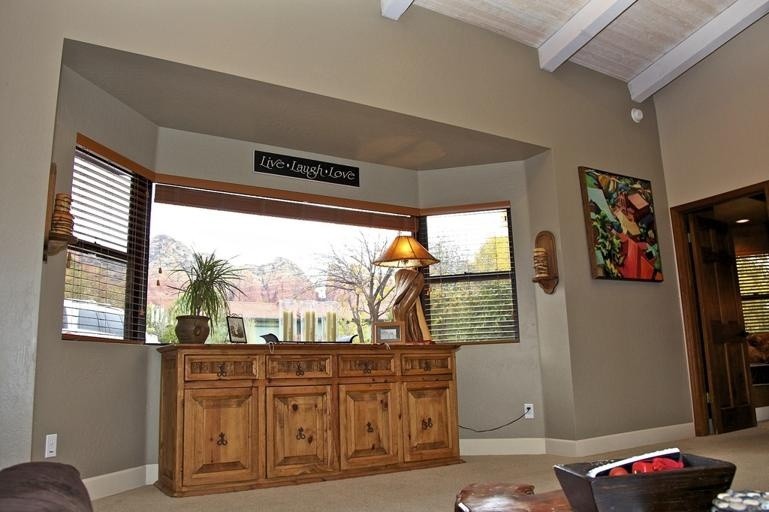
[551,449,738,511]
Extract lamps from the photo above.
[530,229,560,295]
[375,236,441,346]
[44,161,79,262]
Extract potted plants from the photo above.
[165,250,253,344]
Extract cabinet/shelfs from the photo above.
[154,342,467,498]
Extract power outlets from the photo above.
[524,403,535,419]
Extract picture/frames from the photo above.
[577,164,664,283]
[226,316,248,344]
[370,320,406,346]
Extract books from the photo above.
[588,447,681,480]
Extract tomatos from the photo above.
[608,458,685,478]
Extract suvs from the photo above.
[63,297,161,344]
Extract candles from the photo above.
[283,311,336,342]
